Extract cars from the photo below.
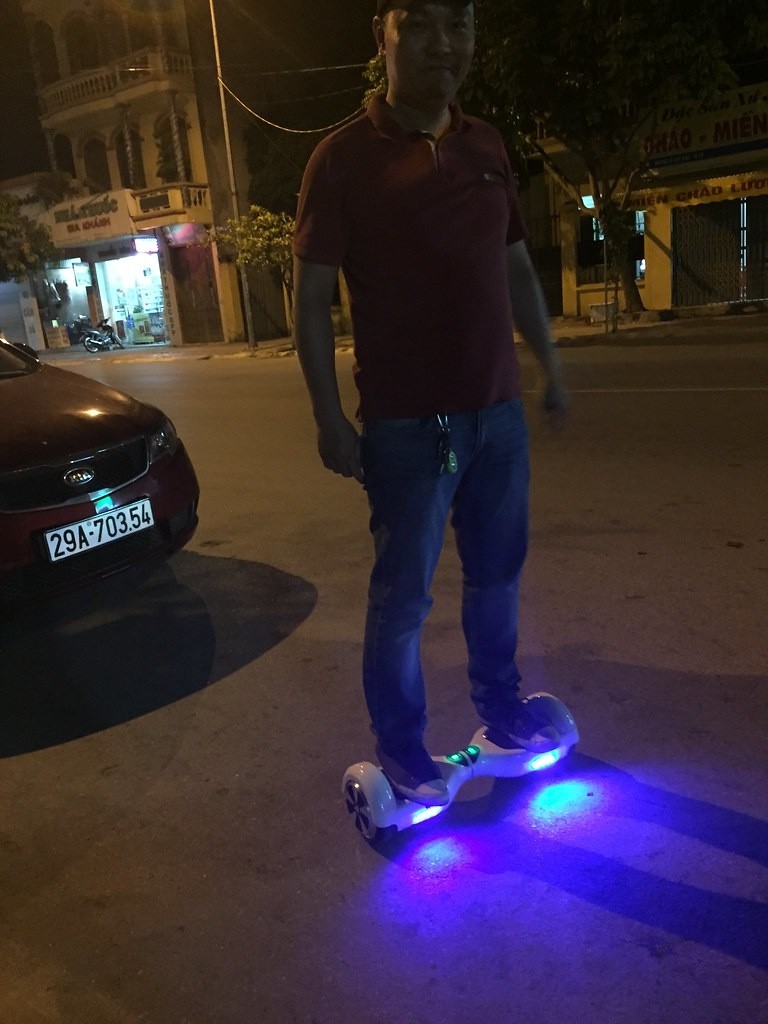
[1,341,202,611]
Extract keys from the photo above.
[434,424,458,476]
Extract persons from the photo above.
[291,0,569,814]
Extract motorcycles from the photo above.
[80,320,126,353]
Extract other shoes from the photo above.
[376,741,449,806]
[478,704,559,751]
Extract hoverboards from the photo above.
[343,694,581,852]
